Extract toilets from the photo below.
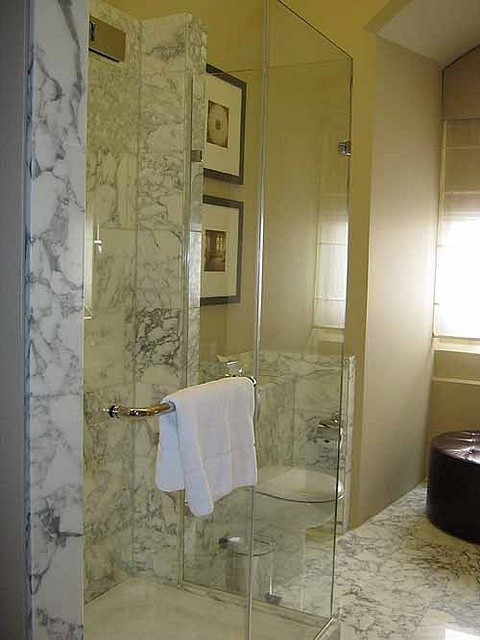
[229,463,345,581]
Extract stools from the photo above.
[426,428,480,543]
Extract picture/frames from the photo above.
[203,62,247,184]
[200,194,244,306]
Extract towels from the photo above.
[154,377,258,518]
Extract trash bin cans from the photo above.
[219,535,277,601]
